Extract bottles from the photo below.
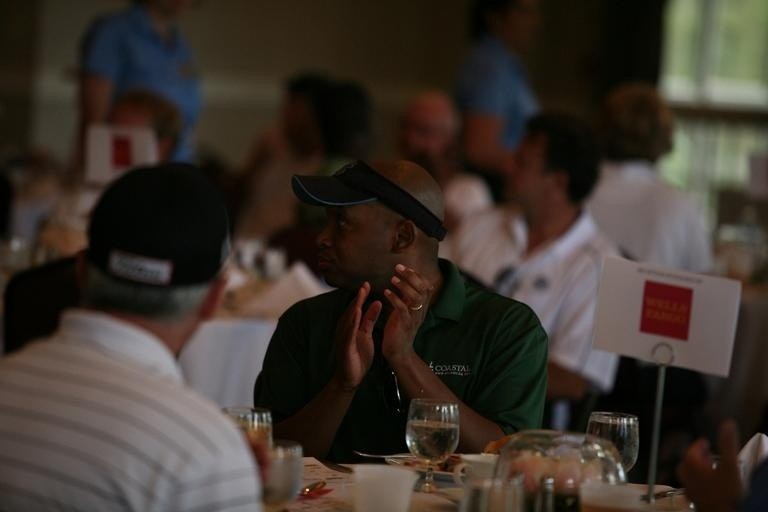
[532,472,574,512]
[457,475,525,512]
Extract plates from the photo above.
[584,483,689,512]
[385,451,467,483]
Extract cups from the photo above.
[353,462,419,511]
[584,411,640,483]
[223,404,303,512]
[461,454,500,479]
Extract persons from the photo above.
[0,0,768,511]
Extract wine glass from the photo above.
[402,397,459,496]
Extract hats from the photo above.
[86,160,233,291]
[291,160,448,243]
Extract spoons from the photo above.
[294,480,325,495]
